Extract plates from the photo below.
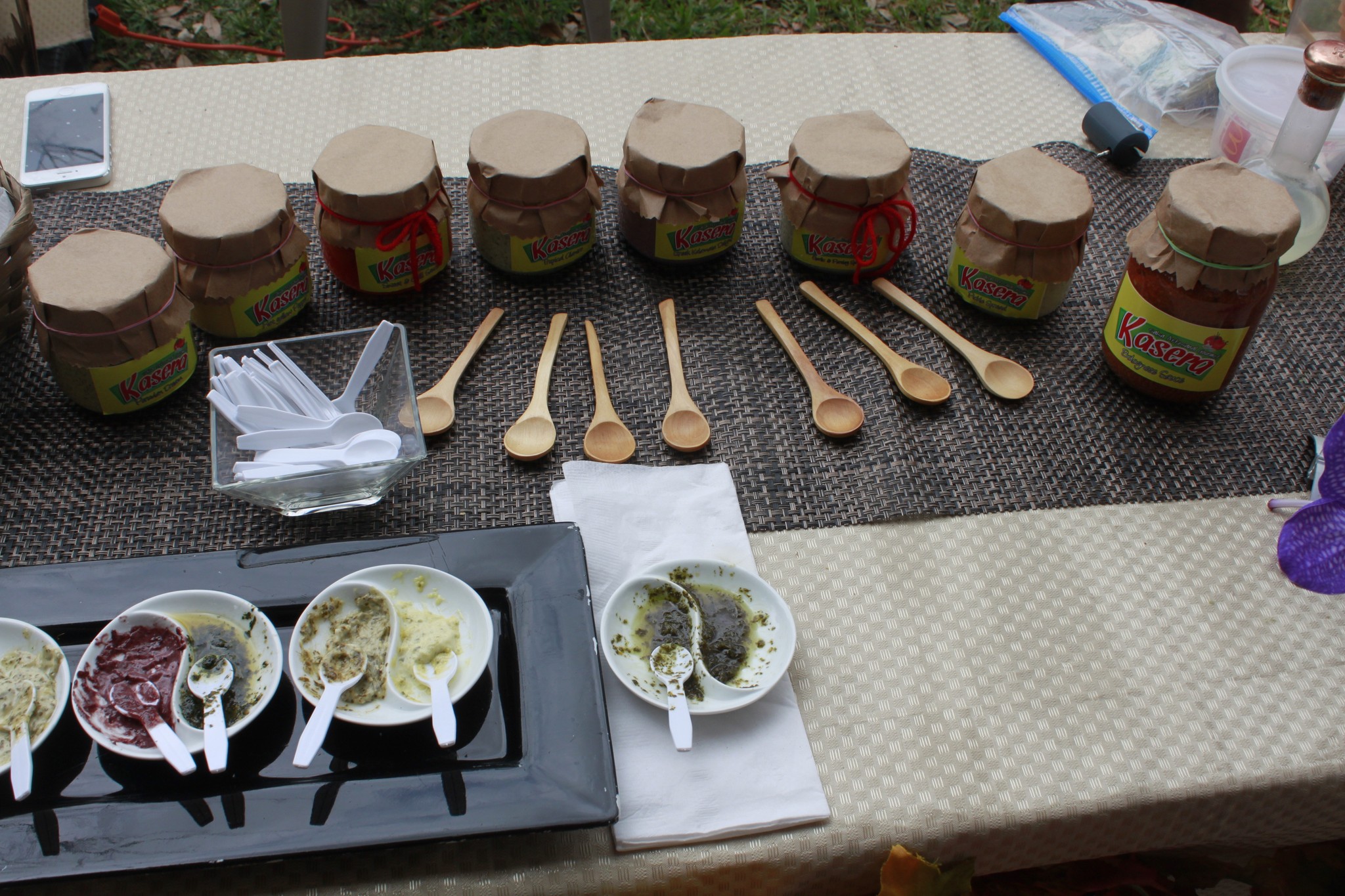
[0,520,622,890]
[602,558,798,716]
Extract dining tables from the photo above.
[0,31,1343,896]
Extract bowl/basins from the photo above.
[190,324,427,518]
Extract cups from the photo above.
[1217,39,1344,193]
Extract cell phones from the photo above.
[18,82,112,195]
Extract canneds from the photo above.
[26,98,1304,423]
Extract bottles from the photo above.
[1236,36,1344,265]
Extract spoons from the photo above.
[757,298,865,434]
[797,279,951,406]
[503,310,571,456]
[0,647,704,802]
[875,270,1035,404]
[205,320,401,494]
[582,318,638,462]
[657,298,712,453]
[402,304,502,433]
